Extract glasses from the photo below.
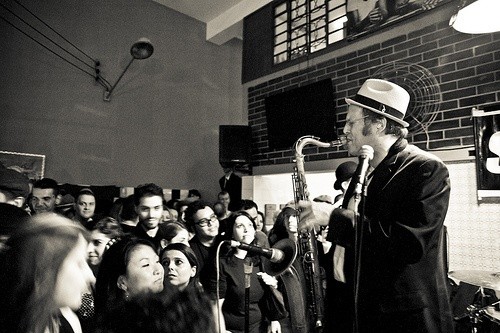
[346,114,369,127]
[195,214,218,228]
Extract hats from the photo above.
[344,79,411,128]
[313,195,332,204]
[334,161,358,190]
[282,199,296,211]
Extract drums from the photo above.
[476,300,500,333]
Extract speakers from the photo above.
[219,125,251,167]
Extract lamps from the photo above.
[102,38,153,102]
[450,0,500,34]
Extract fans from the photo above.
[370,61,442,137]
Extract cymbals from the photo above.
[447,269,500,291]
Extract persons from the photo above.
[0,168,264,277]
[297,79,455,333]
[275,201,310,333]
[158,244,200,305]
[209,211,289,333]
[0,212,96,333]
[92,237,164,333]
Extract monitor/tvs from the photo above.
[265,78,336,151]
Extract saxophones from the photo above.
[261,135,350,333]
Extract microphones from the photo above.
[354,144,374,202]
[226,239,284,263]
